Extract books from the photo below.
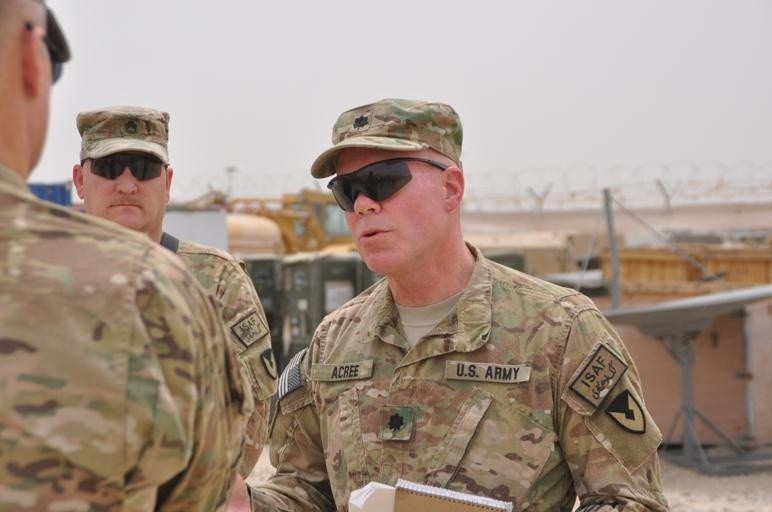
[389,475,515,512]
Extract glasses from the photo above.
[22,22,61,84]
[326,153,448,215]
[87,151,166,182]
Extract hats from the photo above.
[309,95,464,181]
[26,0,71,63]
[72,105,170,167]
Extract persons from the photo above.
[222,96,677,512]
[70,104,278,486]
[0,1,245,512]
